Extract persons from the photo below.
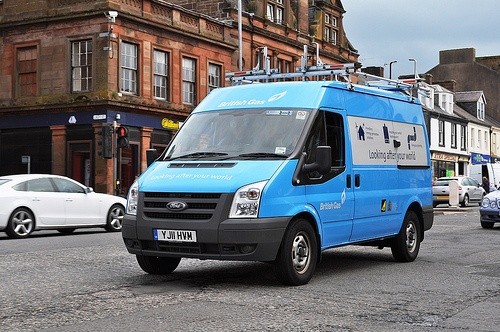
[482,175,490,194]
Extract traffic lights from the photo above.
[116,126,130,150]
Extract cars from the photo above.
[0,173,128,239]
[433,177,487,208]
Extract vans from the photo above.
[121,45,434,286]
